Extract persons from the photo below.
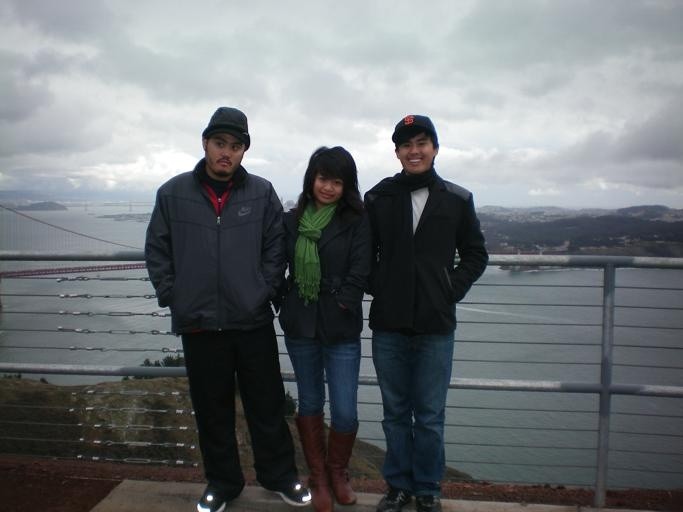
[142,106,314,512]
[271,144,374,511]
[364,113,487,511]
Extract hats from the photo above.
[203,107,250,151]
[392,115,435,142]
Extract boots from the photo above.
[294,415,333,512]
[328,422,362,505]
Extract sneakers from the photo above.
[264,481,312,507]
[377,488,410,512]
[416,495,441,511]
[197,485,227,512]
